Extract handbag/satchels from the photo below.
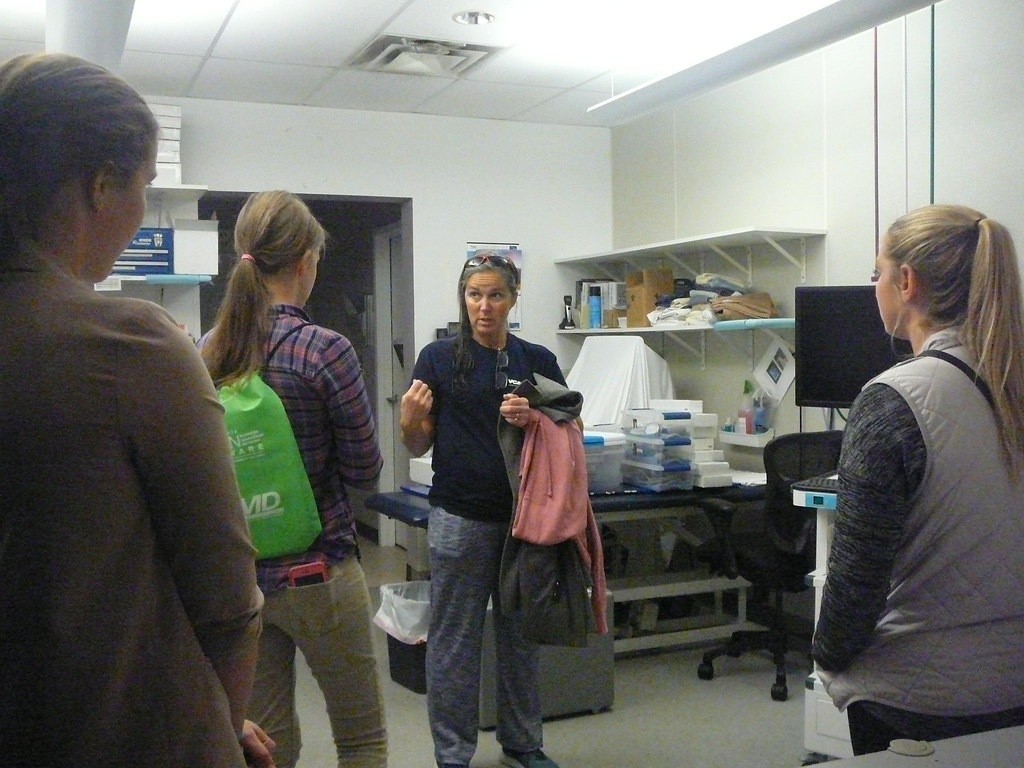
[213,324,322,560]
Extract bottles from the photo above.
[722,395,772,436]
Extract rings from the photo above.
[515,415,520,421]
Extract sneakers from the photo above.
[501,745,559,768]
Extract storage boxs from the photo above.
[604,309,629,329]
[572,433,627,494]
[623,269,674,328]
[479,587,616,729]
[620,410,693,492]
[164,212,219,276]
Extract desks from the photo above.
[364,488,780,672]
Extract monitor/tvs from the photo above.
[794,285,915,409]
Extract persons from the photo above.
[813,203,1023,755]
[406,254,570,768]
[0,53,276,768]
[195,191,389,768]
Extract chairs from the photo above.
[692,430,846,701]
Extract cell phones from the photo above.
[287,562,328,586]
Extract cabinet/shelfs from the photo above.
[555,228,826,335]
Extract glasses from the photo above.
[464,256,514,277]
[494,348,509,389]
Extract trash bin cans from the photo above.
[380,580,432,694]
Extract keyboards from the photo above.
[791,476,838,494]
